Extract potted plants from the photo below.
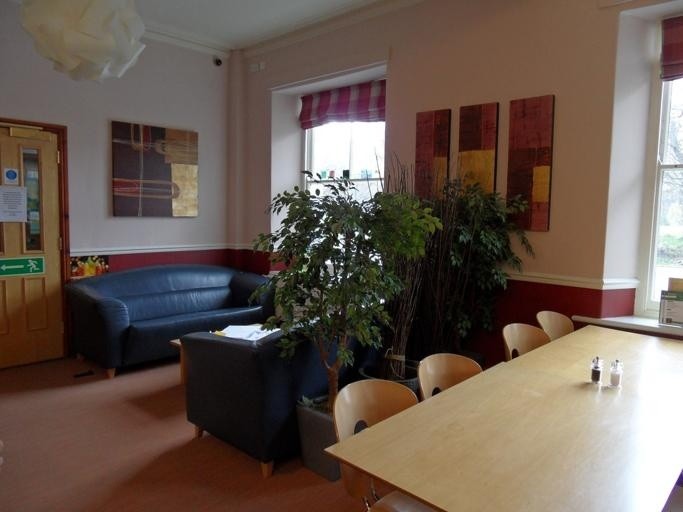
[249,176,443,481]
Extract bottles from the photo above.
[590,356,622,386]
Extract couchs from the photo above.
[67,265,276,378]
[181,298,397,478]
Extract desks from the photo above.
[325,323,683,512]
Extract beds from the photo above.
[169,339,181,348]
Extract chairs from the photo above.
[332,377,418,512]
[503,323,551,360]
[417,353,482,400]
[536,310,574,340]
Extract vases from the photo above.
[358,365,419,394]
